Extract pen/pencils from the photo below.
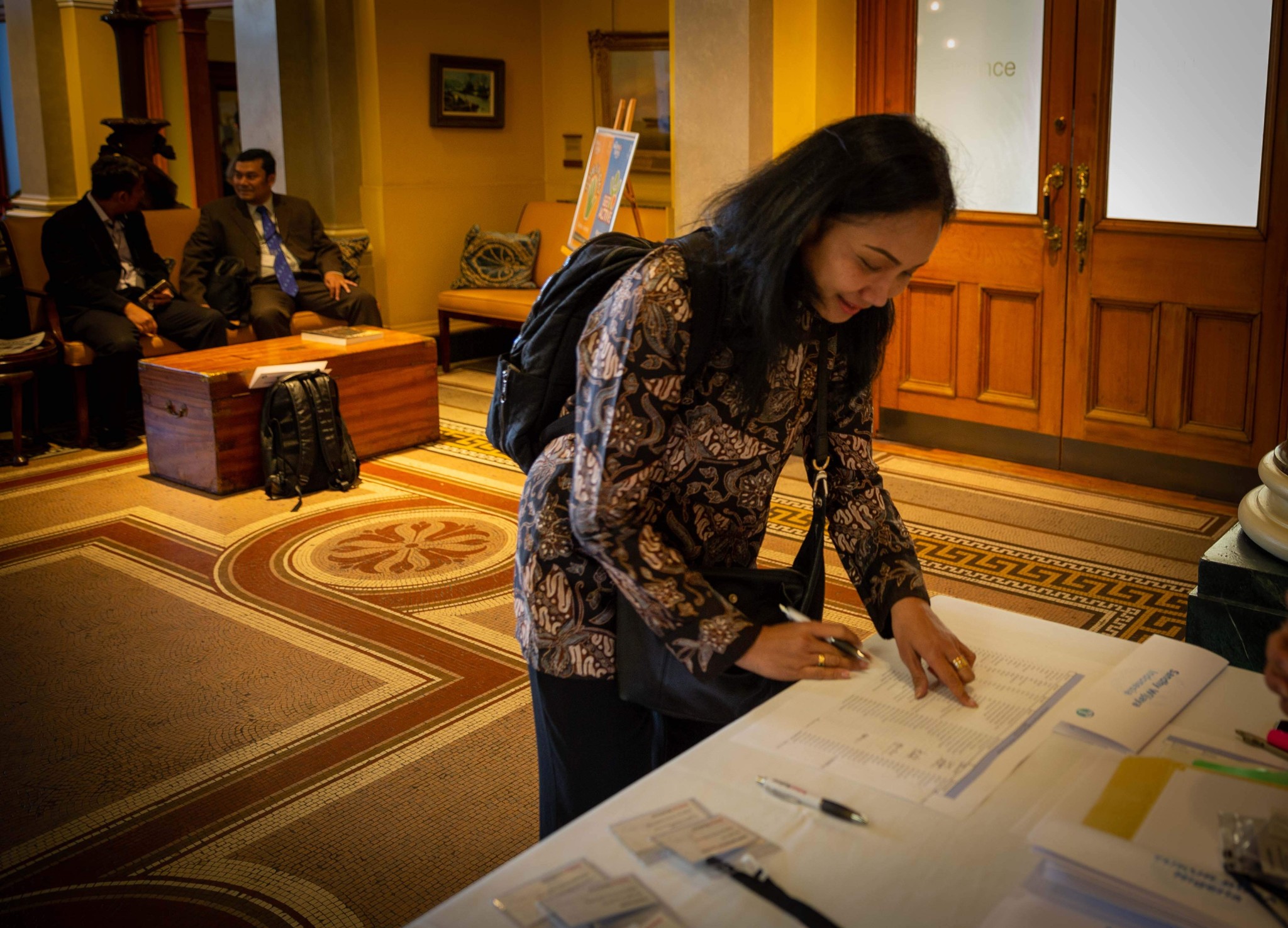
[1235,729,1288,760]
[779,603,871,663]
[755,774,868,824]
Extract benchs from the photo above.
[7,209,350,448]
[435,201,667,373]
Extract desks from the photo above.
[406,587,1288,928]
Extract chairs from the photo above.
[0,235,65,468]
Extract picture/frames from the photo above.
[586,29,670,173]
[429,53,506,130]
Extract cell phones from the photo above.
[139,279,172,305]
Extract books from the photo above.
[300,327,383,346]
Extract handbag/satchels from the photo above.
[613,470,831,728]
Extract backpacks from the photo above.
[486,230,718,479]
[261,370,360,512]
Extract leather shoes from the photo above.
[98,410,125,449]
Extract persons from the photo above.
[179,147,383,343]
[41,156,226,451]
[1264,619,1288,718]
[516,113,978,845]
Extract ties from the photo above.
[256,205,300,299]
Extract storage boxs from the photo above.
[139,324,440,498]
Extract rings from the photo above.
[818,654,825,667]
[953,654,969,672]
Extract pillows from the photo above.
[449,222,540,292]
[323,235,369,282]
